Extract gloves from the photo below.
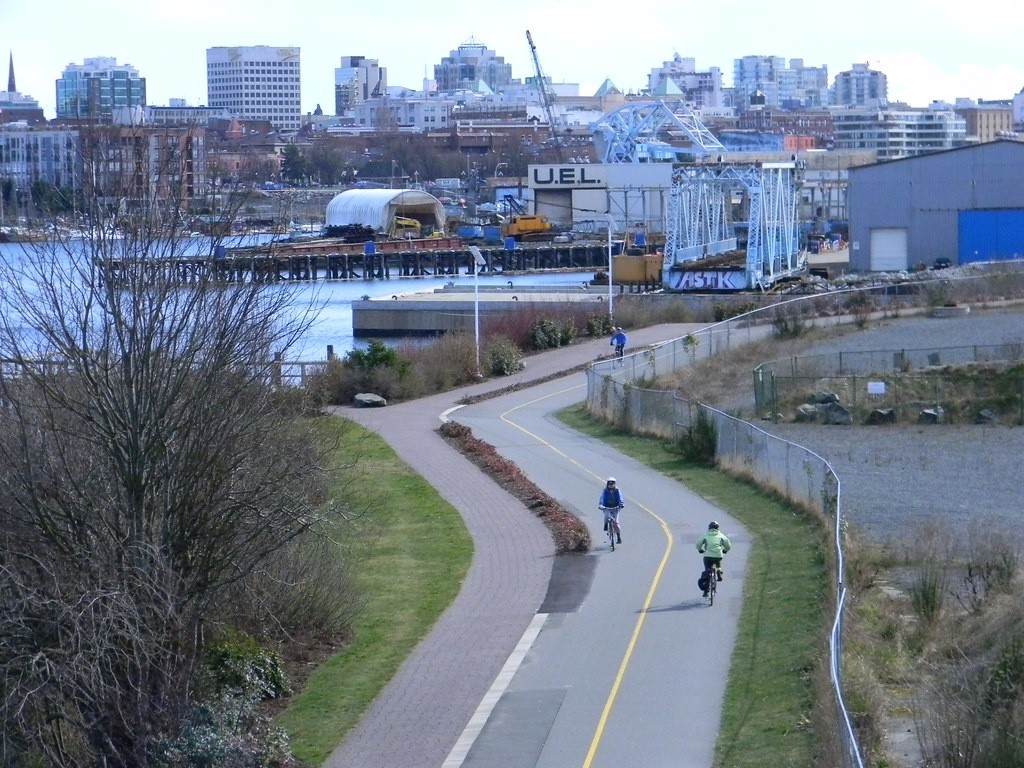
[610,342,614,345]
[723,550,727,553]
[700,550,703,553]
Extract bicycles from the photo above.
[611,343,624,369]
[699,549,728,605]
[598,505,626,551]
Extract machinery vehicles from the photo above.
[500,194,549,242]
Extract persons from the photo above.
[696,521,731,598]
[598,477,624,544]
[610,327,628,366]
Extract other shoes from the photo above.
[603,523,608,531]
[615,533,622,544]
[715,571,723,582]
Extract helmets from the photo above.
[617,327,622,330]
[709,520,718,529]
[606,477,616,490]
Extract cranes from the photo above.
[524,29,588,165]
